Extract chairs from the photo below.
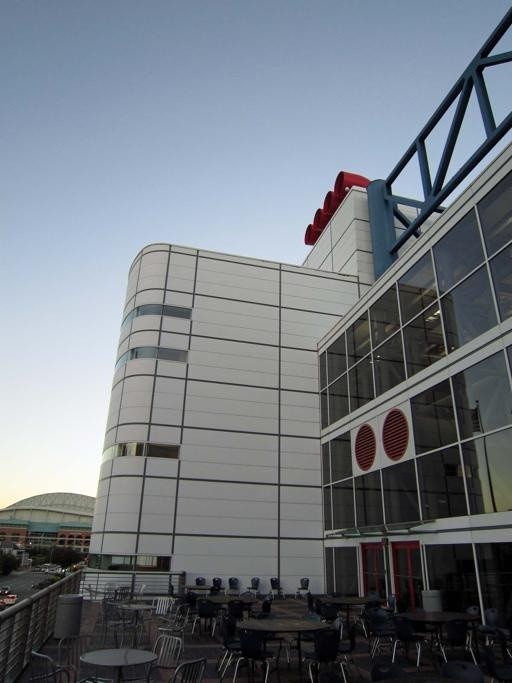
[170,579,272,638]
[216,617,364,683]
[296,578,310,600]
[305,589,397,641]
[369,606,512,681]
[32,628,206,682]
[98,582,174,647]
[270,578,286,600]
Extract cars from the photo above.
[33,563,65,577]
[0,586,18,610]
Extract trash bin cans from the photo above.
[54,594,83,639]
[422,590,443,629]
